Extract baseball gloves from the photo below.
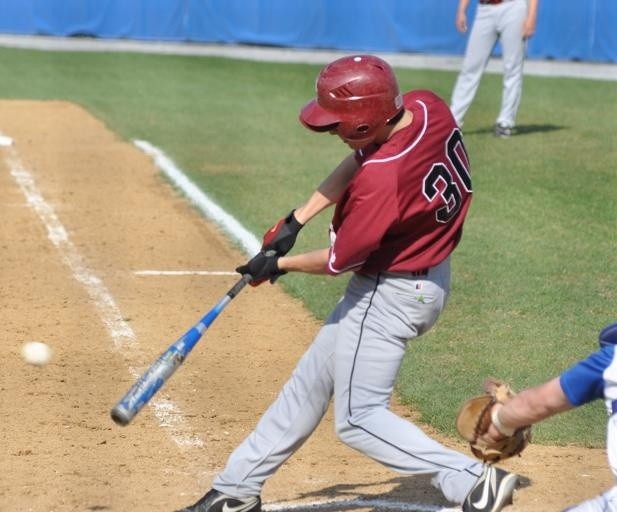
[457,381,532,464]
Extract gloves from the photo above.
[263,208,301,257]
[234,251,287,286]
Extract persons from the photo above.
[176,54,518,511]
[451,0,538,136]
[455,323,617,511]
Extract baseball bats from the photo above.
[109,249,277,427]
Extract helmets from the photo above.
[299,53,404,143]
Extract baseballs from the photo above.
[25,342,49,366]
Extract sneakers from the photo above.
[175,487,262,512]
[462,463,519,512]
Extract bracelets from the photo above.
[491,407,513,437]
